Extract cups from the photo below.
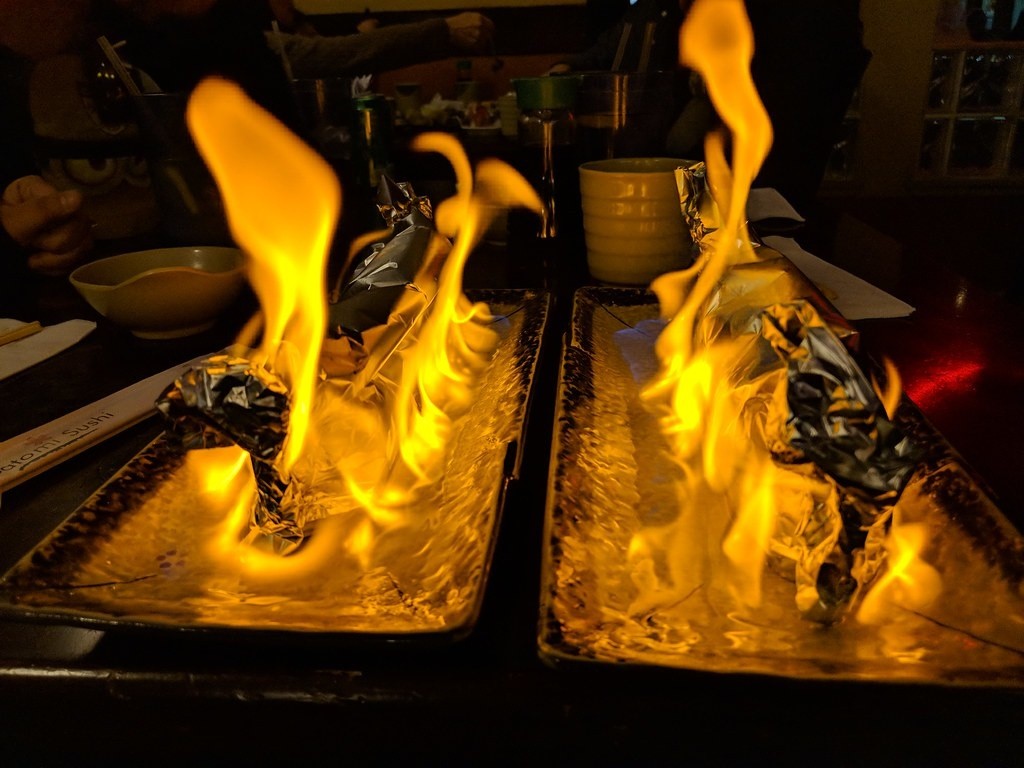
[580,155,702,285]
[497,96,519,134]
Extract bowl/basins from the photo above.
[512,74,583,113]
[462,124,500,139]
[68,245,251,340]
[580,70,666,116]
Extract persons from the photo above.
[0,171,94,317]
[453,62,498,101]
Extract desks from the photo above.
[0,211,1024,768]
[321,115,646,177]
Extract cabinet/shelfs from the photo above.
[917,40,1024,186]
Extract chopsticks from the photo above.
[0,320,42,347]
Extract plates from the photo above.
[0,292,549,633]
[539,289,1024,686]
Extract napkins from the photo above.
[0,316,98,380]
